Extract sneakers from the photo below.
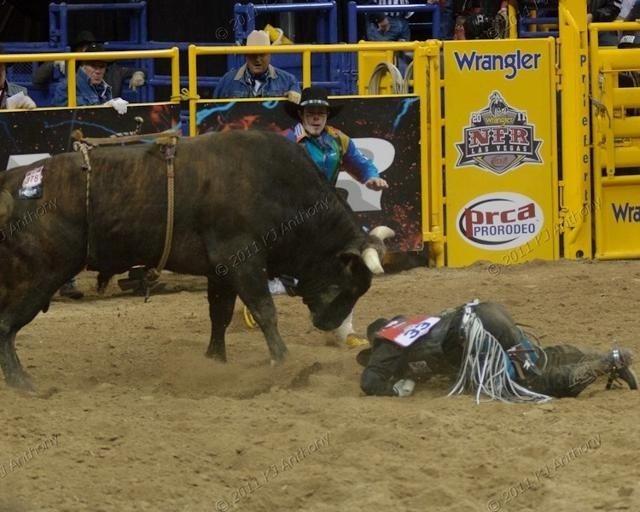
[335,330,369,348]
[244,305,256,328]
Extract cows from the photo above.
[0,128,396,392]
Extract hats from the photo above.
[86,47,113,66]
[247,30,270,45]
[284,88,343,121]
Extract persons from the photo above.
[355,298,638,398]
[242,84,392,351]
[355,0,639,117]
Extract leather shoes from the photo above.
[606,349,637,389]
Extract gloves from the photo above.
[18,96,36,109]
[393,379,415,396]
[104,98,128,115]
